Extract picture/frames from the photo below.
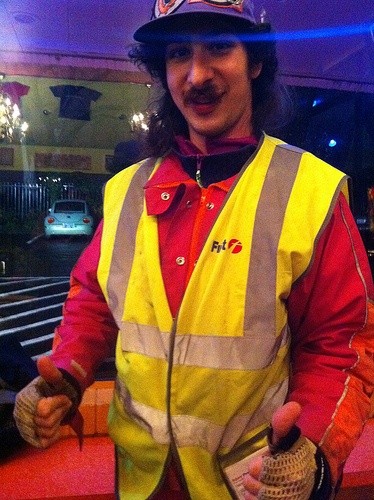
[105,154,113,170]
[0,147,14,166]
[36,151,92,170]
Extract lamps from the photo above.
[0,71,29,144]
[307,88,317,109]
[328,138,337,147]
[129,83,164,133]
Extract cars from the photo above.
[42,198,96,240]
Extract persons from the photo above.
[13,0,374,500]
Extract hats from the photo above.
[133,0,258,44]
